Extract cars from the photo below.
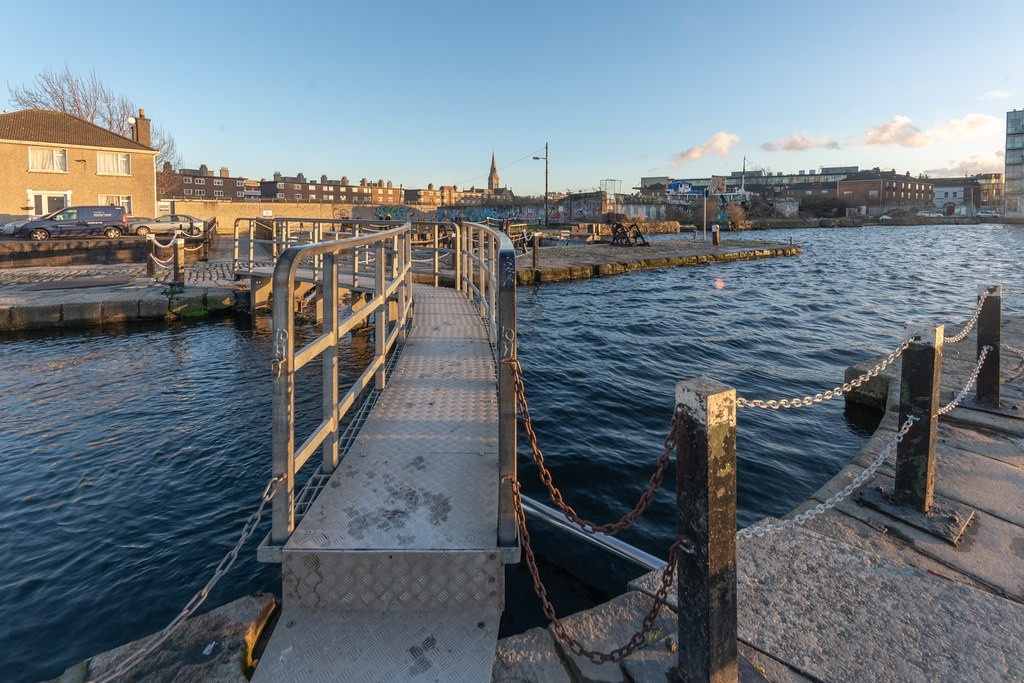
[916,211,943,218]
[123,214,207,236]
[976,209,1001,218]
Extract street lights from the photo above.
[533,142,549,226]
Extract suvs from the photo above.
[3,204,127,239]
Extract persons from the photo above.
[375,212,392,230]
